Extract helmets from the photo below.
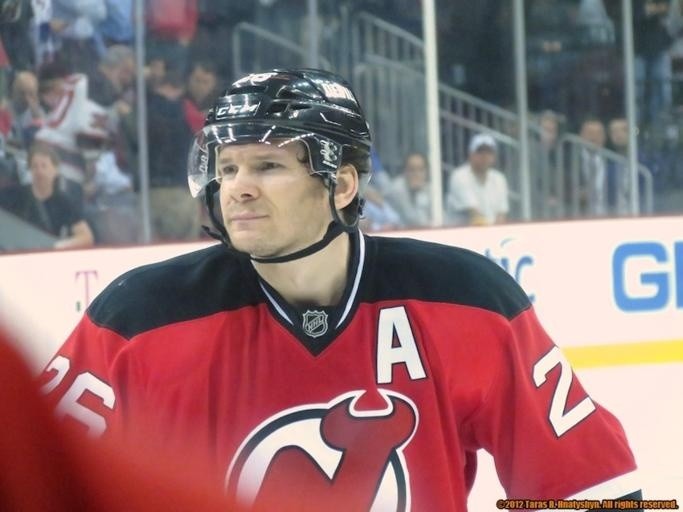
[185,65,374,202]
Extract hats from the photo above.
[467,133,497,153]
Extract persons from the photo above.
[34,66,645,512]
[3,1,682,250]
